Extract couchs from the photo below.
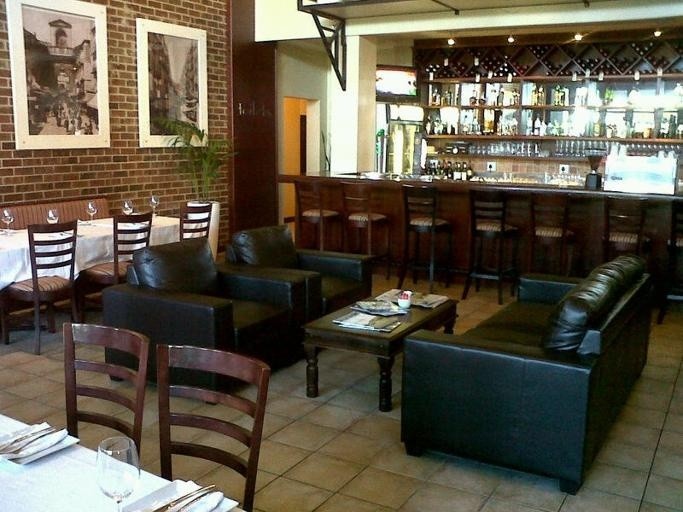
[400,254,655,496]
[231,226,374,312]
[106,236,308,402]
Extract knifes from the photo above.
[153,484,215,512]
[0,422,79,467]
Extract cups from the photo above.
[469,141,540,158]
[395,289,412,308]
[46,209,59,224]
[122,201,136,215]
[552,139,681,160]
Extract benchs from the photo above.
[0,194,112,231]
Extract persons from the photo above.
[51,98,101,135]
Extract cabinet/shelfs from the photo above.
[419,78,681,162]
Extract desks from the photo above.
[303,288,457,413]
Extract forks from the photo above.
[1,426,65,456]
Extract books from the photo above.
[330,286,451,332]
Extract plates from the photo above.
[120,477,240,512]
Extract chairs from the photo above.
[177,204,214,242]
[463,190,517,302]
[603,194,650,263]
[78,211,155,318]
[4,218,85,353]
[293,179,342,251]
[529,188,575,277]
[400,185,450,291]
[62,319,149,467]
[156,342,271,506]
[659,200,682,325]
[342,183,391,258]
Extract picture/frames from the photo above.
[4,0,110,153]
[134,18,210,149]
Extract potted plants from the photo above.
[151,111,239,264]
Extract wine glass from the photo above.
[95,435,139,512]
[85,200,98,226]
[1,207,19,235]
[147,193,162,219]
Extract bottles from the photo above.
[425,159,473,180]
[422,40,682,138]
